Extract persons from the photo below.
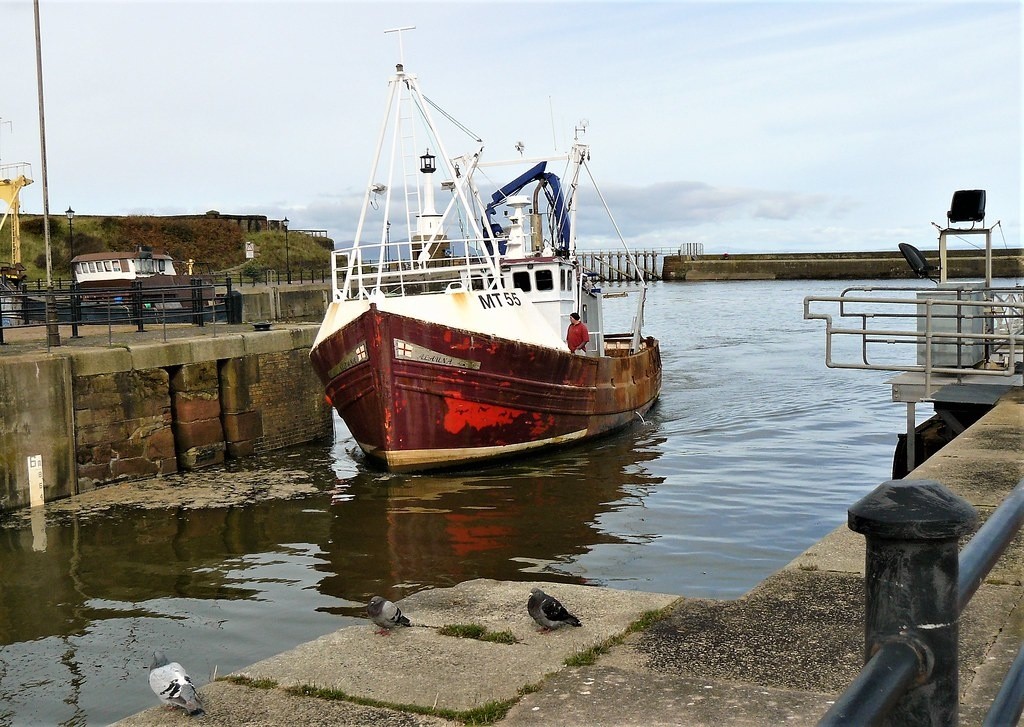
[566,313,589,354]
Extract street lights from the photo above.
[386,222,391,271]
[66,206,82,338]
[282,216,291,284]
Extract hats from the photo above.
[570,312,580,320]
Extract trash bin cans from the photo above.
[224,290,243,324]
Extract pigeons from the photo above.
[527,587,582,635]
[367,596,412,637]
[147,650,205,717]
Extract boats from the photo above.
[309,26,663,471]
[73,248,218,307]
